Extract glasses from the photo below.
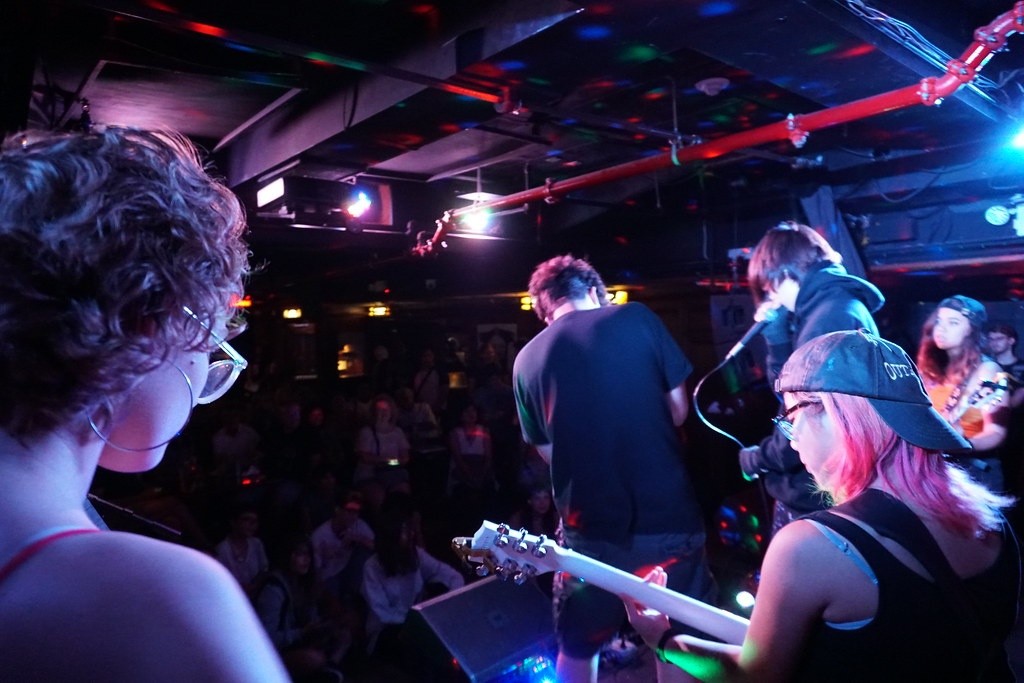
[181,304,247,405]
[988,336,1005,341]
[772,401,821,442]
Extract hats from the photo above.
[775,329,973,451]
[939,295,987,330]
[989,325,1018,347]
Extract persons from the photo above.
[988,323,1022,384]
[509,258,709,683]
[738,224,883,534]
[157,323,557,657]
[0,120,296,683]
[617,327,1024,683]
[916,295,1010,499]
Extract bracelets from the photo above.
[654,628,682,664]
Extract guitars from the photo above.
[450,515,752,648]
